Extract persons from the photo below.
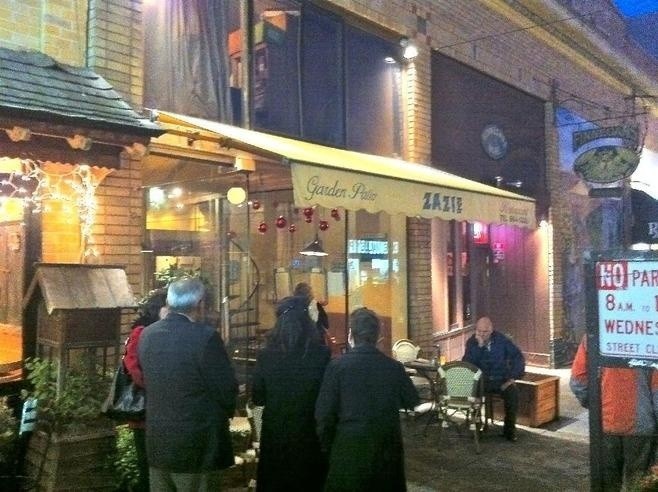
[295,281,329,345]
[122,288,170,492]
[568,332,658,492]
[314,306,420,492]
[462,317,525,442]
[136,276,238,491]
[250,294,332,492]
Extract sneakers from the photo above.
[504,426,518,441]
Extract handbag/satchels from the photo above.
[98,337,146,421]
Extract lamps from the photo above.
[5,126,146,160]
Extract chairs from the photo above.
[392,339,487,453]
